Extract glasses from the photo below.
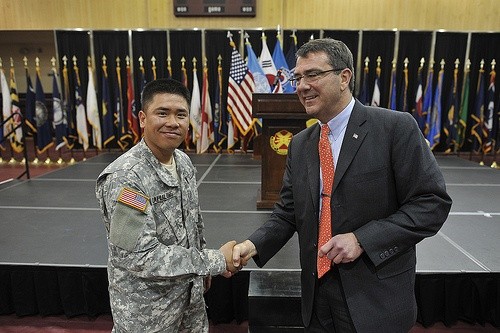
[289,67,352,87]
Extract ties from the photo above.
[317,125,334,279]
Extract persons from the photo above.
[223,38,453,333]
[95,77,248,333]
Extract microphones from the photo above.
[273,69,282,85]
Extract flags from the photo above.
[358,56,500,154]
[225,30,298,155]
[0,55,227,153]
